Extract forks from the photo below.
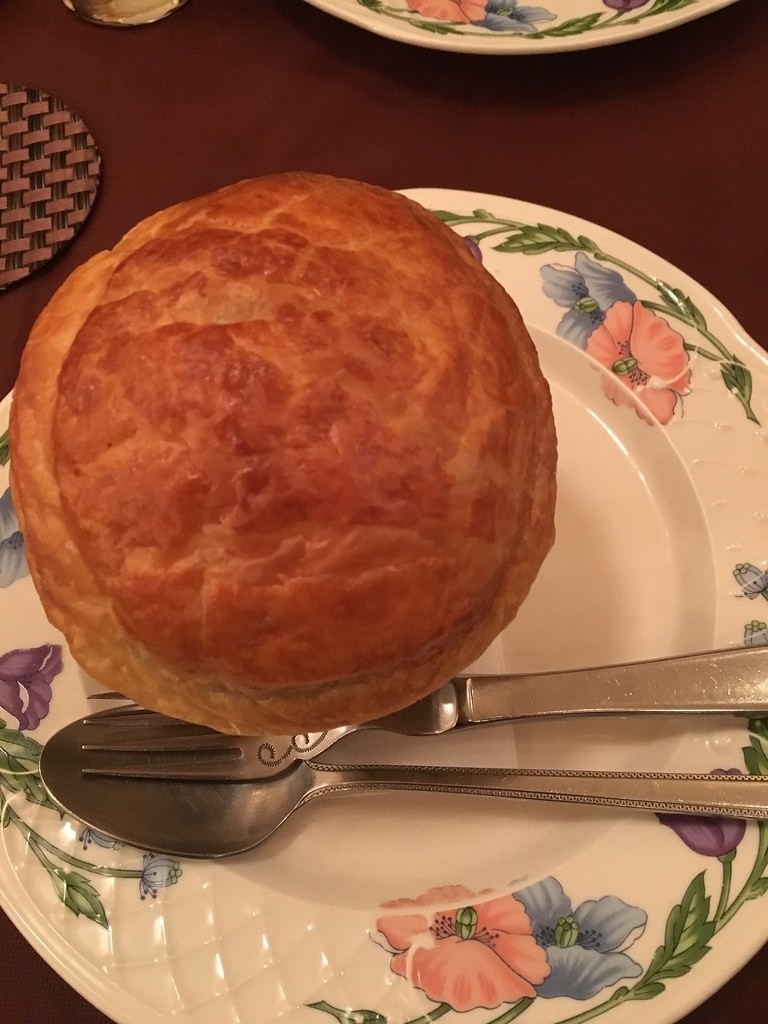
[78,644,768,792]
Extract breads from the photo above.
[8,172,558,735]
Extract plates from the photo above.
[309,0,747,61]
[0,188,768,1023]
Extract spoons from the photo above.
[33,699,767,862]
